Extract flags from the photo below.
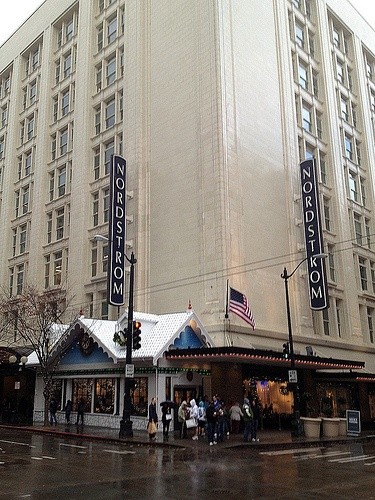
[229,287,255,331]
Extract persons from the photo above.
[75,397,84,425]
[177,394,260,446]
[49,397,58,426]
[147,397,158,440]
[263,403,273,416]
[65,400,71,423]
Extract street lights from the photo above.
[280,252,329,438]
[9,355,27,424]
[93,234,133,438]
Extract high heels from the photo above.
[192,435,198,440]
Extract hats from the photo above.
[199,401,204,406]
[215,393,220,397]
[244,398,249,403]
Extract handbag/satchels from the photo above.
[148,418,158,434]
[186,419,197,428]
[166,414,172,420]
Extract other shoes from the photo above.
[210,441,217,445]
[252,438,259,442]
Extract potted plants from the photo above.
[300,392,347,438]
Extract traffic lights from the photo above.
[132,321,141,350]
[283,343,290,359]
